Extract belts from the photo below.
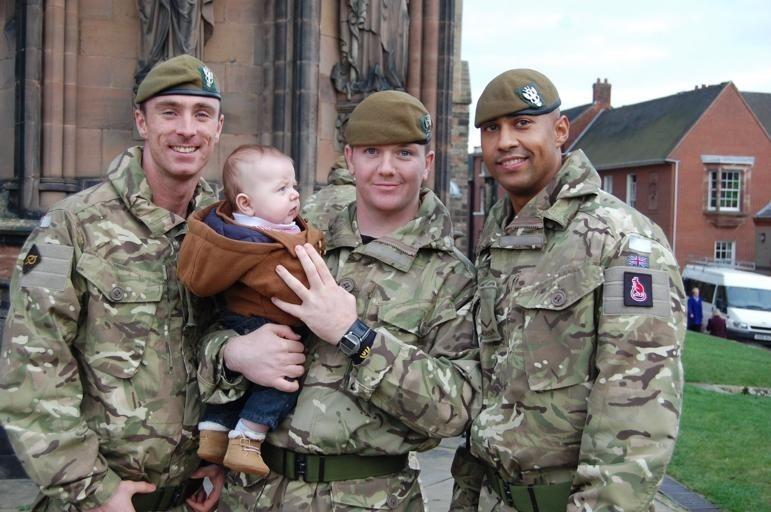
[482,467,575,511]
[133,476,204,511]
[263,444,408,481]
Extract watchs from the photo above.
[336,318,371,359]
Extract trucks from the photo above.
[679,255,771,344]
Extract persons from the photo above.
[444,66,689,511]
[299,154,357,245]
[687,286,703,332]
[0,52,228,512]
[194,89,479,510]
[176,143,329,479]
[706,308,727,338]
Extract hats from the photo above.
[133,55,222,104]
[475,69,561,127]
[346,90,433,145]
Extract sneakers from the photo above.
[225,439,270,475]
[197,429,230,465]
